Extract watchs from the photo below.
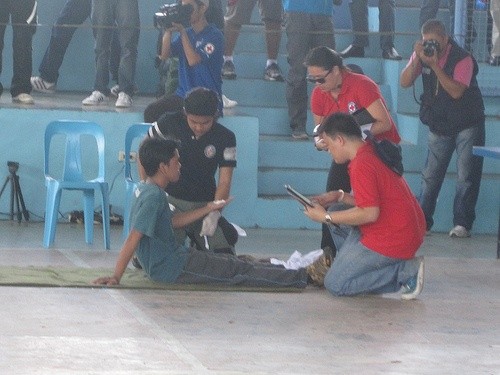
[324,212,332,224]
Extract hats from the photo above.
[182,86,220,116]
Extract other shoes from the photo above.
[291,130,308,140]
[489,56,500,66]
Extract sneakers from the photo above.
[264,62,283,81]
[13,92,35,104]
[306,245,334,290]
[449,225,472,238]
[81,89,110,105]
[30,76,57,93]
[400,256,425,301]
[221,58,237,80]
[110,83,120,98]
[114,91,133,108]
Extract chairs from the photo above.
[123,123,154,238]
[42,120,111,250]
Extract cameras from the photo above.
[154,3,194,29]
[422,40,441,58]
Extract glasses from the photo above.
[306,68,333,84]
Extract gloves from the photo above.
[199,210,221,238]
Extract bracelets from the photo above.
[338,189,344,201]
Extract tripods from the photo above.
[0,173,30,221]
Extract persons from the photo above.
[420,0,500,66]
[1,0,141,109]
[304,47,403,257]
[401,19,484,238]
[303,112,427,300]
[136,88,237,255]
[341,0,401,60]
[281,0,343,139]
[144,0,282,126]
[92,136,334,288]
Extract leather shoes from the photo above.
[381,46,403,61]
[340,44,365,58]
